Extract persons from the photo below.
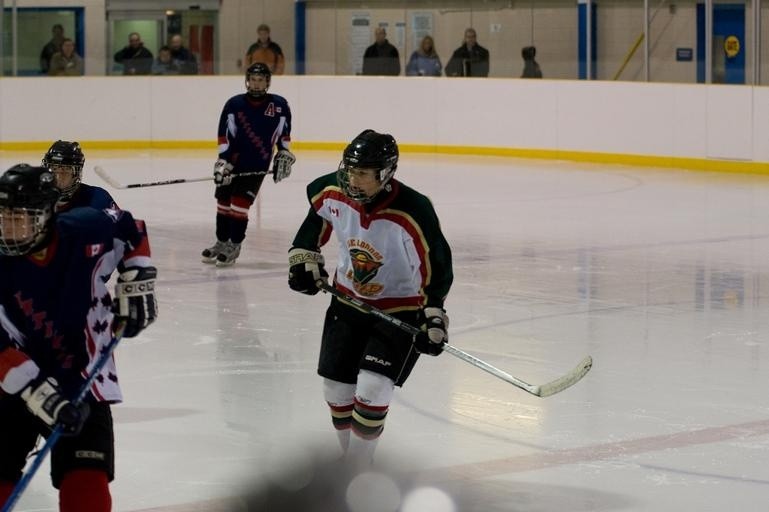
[290,128,453,466]
[41,139,119,212]
[362,27,400,76]
[519,46,542,78]
[444,28,489,77]
[40,24,198,75]
[244,24,284,76]
[201,63,296,261]
[0,161,158,512]
[406,36,442,76]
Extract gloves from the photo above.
[214,159,233,187]
[21,375,90,435]
[114,267,157,338]
[273,150,296,183]
[288,248,329,295]
[414,307,448,355]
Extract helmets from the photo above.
[245,63,271,98]
[338,130,398,202]
[0,140,84,257]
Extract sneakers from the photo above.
[202,243,241,262]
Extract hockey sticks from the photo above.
[318,282,592,397]
[93,166,274,189]
